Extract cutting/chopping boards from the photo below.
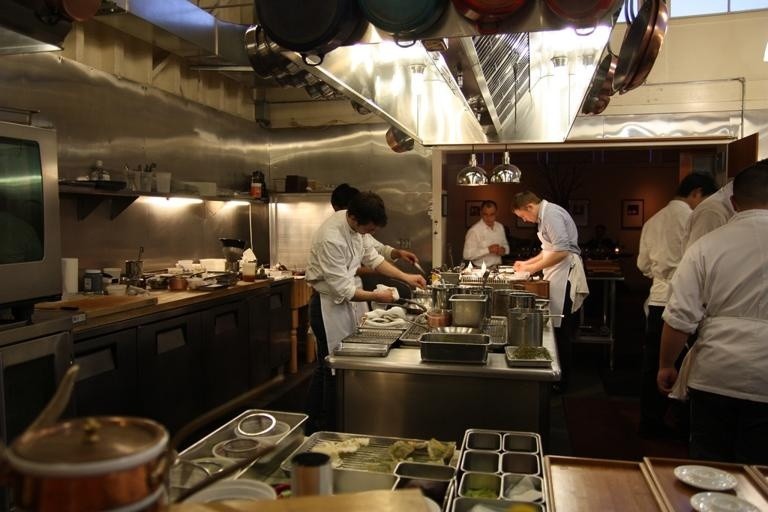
[31,288,159,324]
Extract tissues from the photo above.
[237,248,256,270]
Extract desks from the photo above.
[579,274,624,370]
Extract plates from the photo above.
[688,488,760,511]
[674,463,738,491]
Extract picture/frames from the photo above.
[567,198,589,227]
[620,199,644,230]
[516,216,537,228]
[465,200,487,229]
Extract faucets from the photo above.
[255,258,270,279]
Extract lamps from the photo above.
[455,145,488,187]
[489,145,521,183]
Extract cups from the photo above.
[127,169,172,193]
[241,262,258,281]
[250,182,262,199]
[289,451,333,498]
[61,256,145,293]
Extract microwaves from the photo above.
[0,119,61,303]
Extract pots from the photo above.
[2,414,171,512]
[388,277,492,312]
[255,0,623,67]
[493,289,566,346]
[582,0,669,114]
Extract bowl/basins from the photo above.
[145,271,206,292]
[432,326,482,334]
[188,412,292,478]
[244,21,373,114]
[385,124,414,153]
[180,257,227,271]
[192,478,278,501]
[425,308,452,327]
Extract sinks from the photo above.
[264,268,306,280]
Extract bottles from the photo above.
[85,158,112,181]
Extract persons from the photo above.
[655,155,767,466]
[505,190,584,392]
[680,168,754,266]
[312,190,428,434]
[461,200,511,268]
[633,170,723,441]
[328,183,423,326]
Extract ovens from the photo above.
[0,330,74,419]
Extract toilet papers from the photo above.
[61,258,79,297]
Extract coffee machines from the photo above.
[219,237,246,272]
